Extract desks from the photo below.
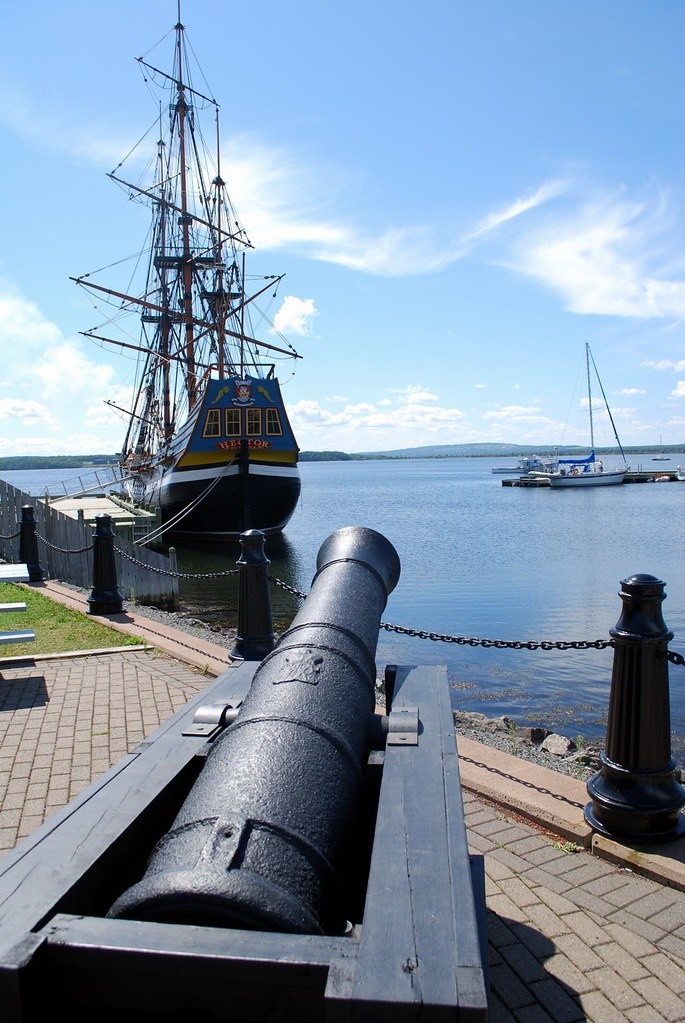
[0,564,30,581]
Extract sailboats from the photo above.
[69,0,303,539]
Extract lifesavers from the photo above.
[574,468,578,474]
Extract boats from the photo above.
[491,342,685,487]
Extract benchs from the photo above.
[0,602,26,611]
[0,630,37,645]
